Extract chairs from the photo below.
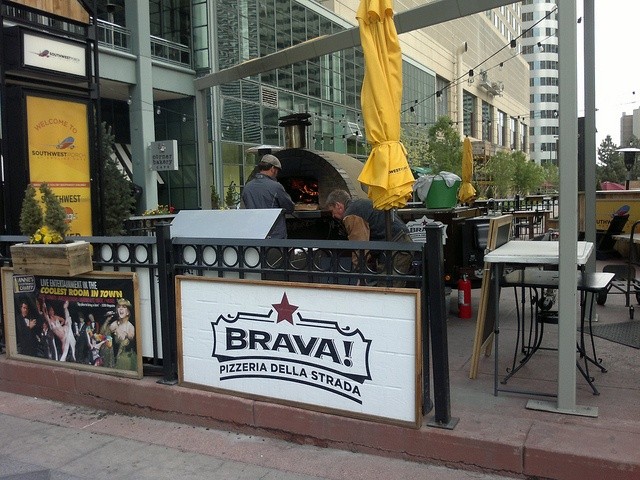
[579,215,629,260]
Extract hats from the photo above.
[261,154,282,171]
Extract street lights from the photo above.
[614,147,640,190]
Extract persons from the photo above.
[16,302,38,357]
[326,190,415,288]
[88,313,100,333]
[36,321,55,360]
[100,299,135,372]
[74,312,87,364]
[85,330,109,367]
[41,297,72,362]
[240,154,295,282]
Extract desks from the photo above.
[395,206,469,225]
[126,214,177,234]
[482,240,592,394]
[503,270,614,395]
[506,210,550,233]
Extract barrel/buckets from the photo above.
[426,178,463,209]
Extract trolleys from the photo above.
[596,222,639,306]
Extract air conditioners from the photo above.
[346,122,362,140]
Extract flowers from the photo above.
[19,184,70,244]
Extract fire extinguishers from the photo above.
[458,272,472,319]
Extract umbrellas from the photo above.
[356,0,414,286]
[458,132,477,208]
